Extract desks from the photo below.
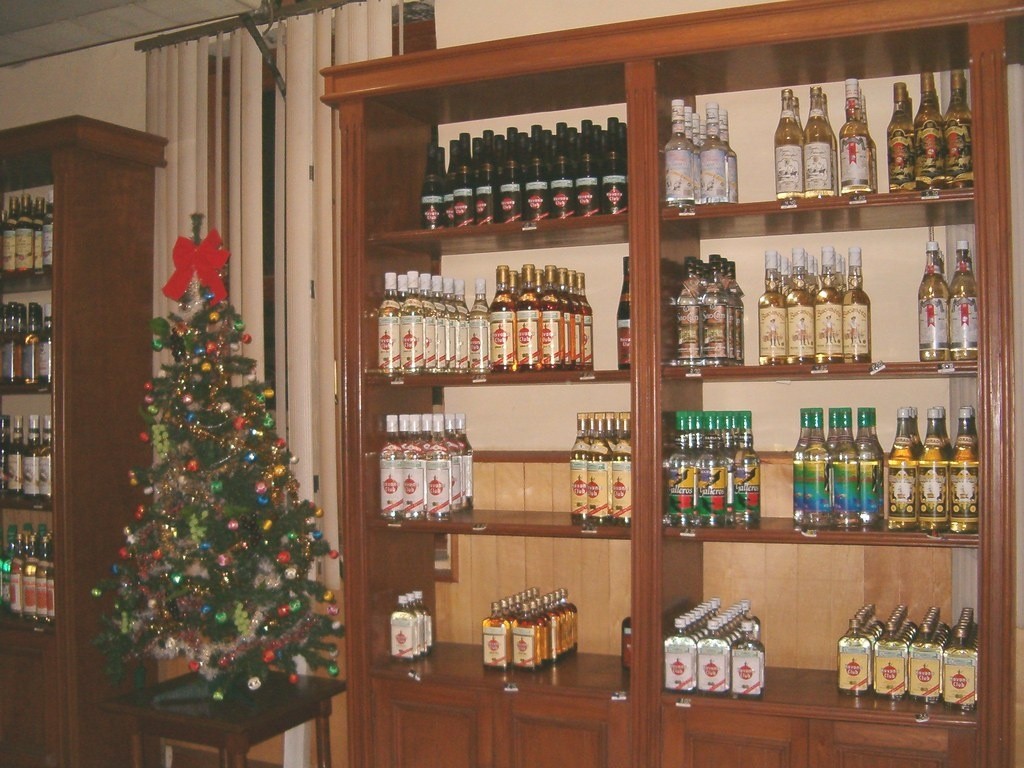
[99,671,346,768]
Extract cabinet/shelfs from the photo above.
[320,1,1024,767]
[0,115,168,768]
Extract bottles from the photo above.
[677,243,979,363]
[0,525,57,624]
[665,599,977,715]
[0,415,52,498]
[420,117,629,228]
[667,67,975,204]
[0,195,54,272]
[665,408,979,534]
[2,300,52,382]
[375,256,632,375]
[392,589,633,675]
[380,412,632,523]
